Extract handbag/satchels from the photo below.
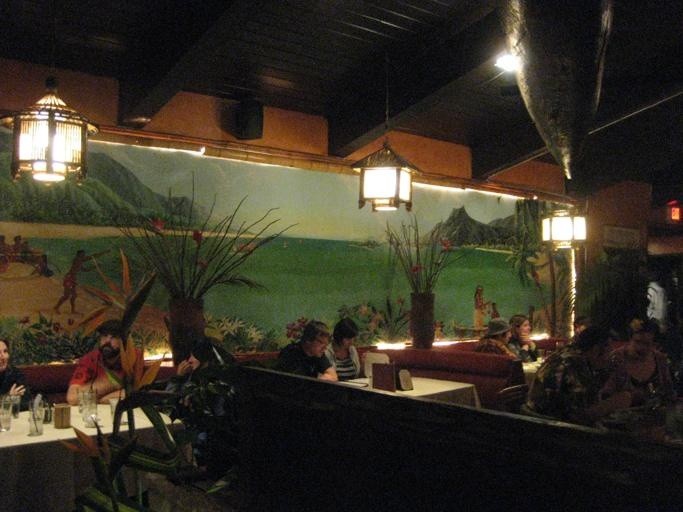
[363,351,390,378]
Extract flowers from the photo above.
[110,170,300,300]
[384,213,480,293]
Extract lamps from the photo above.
[664,200,683,225]
[541,167,587,251]
[0,1,98,185]
[348,48,426,212]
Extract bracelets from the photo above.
[89,379,96,398]
[117,389,123,402]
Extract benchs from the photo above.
[14,366,179,404]
[228,337,569,406]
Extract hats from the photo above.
[487,318,511,336]
[95,319,125,335]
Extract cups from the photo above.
[52,404,71,429]
[26,408,46,436]
[77,386,97,427]
[109,397,127,425]
[0,399,13,432]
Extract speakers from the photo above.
[241,100,262,139]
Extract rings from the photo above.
[18,390,22,394]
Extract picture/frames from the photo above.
[0,124,577,396]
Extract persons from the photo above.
[473,317,518,363]
[572,315,589,334]
[0,235,53,277]
[0,337,34,411]
[54,247,114,314]
[507,313,539,362]
[656,321,675,362]
[157,336,241,490]
[610,317,677,416]
[631,400,678,445]
[528,305,536,321]
[66,319,143,405]
[491,303,500,319]
[276,319,338,384]
[324,317,360,381]
[471,285,491,327]
[524,326,642,423]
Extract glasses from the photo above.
[316,338,329,348]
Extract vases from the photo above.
[168,300,206,366]
[411,295,437,348]
